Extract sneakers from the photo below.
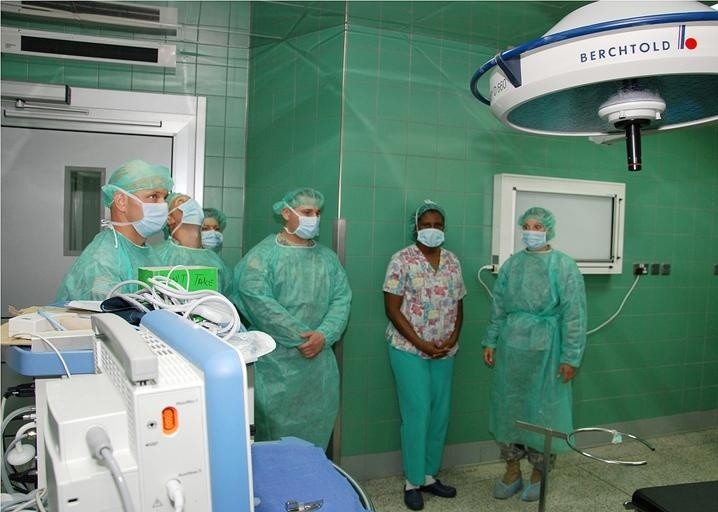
[493,476,523,499]
[421,478,460,498]
[522,480,542,501]
[404,484,424,511]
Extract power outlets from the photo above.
[632,262,650,277]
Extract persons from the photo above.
[232,187,352,458]
[149,193,231,326]
[480,205,586,502]
[55,159,172,302]
[201,207,227,256]
[382,200,467,512]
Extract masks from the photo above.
[199,230,224,250]
[295,216,319,240]
[416,228,445,248]
[521,230,547,251]
[130,202,169,239]
[176,197,206,226]
